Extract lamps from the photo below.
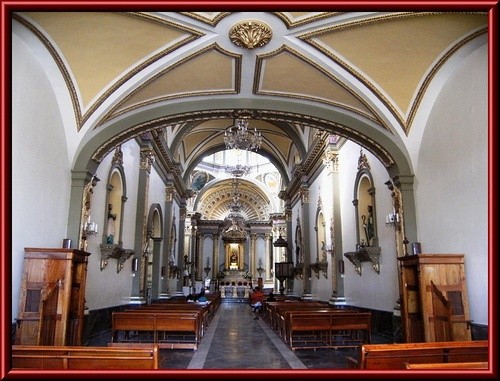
[257,266,263,276]
[223,116,262,152]
[204,266,211,277]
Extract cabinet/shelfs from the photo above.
[14,247,92,347]
[397,253,472,343]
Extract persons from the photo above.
[250,287,263,320]
[197,292,207,302]
[186,294,195,302]
[267,292,276,301]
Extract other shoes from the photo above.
[253,317,259,320]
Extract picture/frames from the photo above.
[0,0,500,380]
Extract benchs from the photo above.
[263,295,488,369]
[12,292,221,369]
